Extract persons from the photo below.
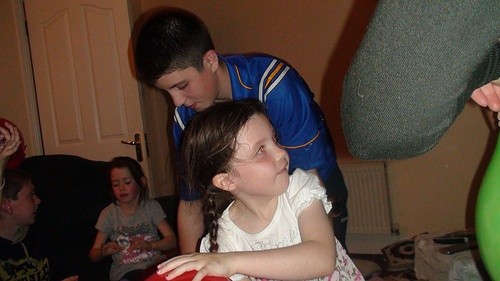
[340,0,500,160]
[0,122,79,281]
[134,9,366,281]
[89,156,176,281]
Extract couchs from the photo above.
[10,154,180,281]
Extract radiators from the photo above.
[335,159,400,237]
